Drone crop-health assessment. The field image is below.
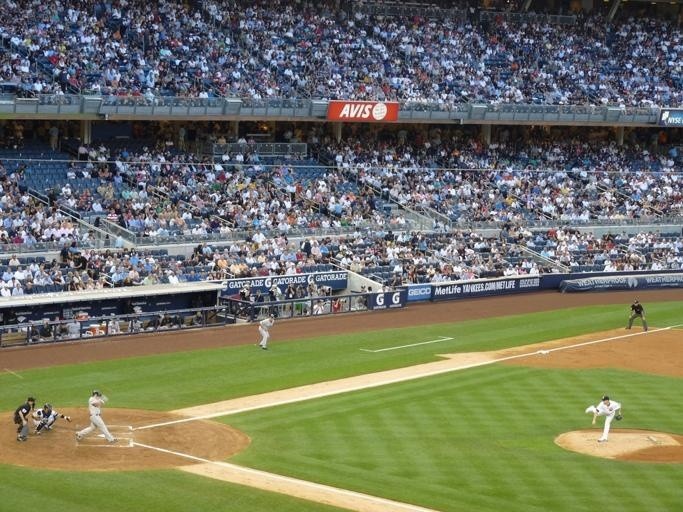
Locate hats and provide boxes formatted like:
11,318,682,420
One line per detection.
602,396,609,400
634,299,639,303
271,314,275,318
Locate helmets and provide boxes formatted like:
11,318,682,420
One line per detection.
93,390,101,395
28,398,35,401
44,403,52,415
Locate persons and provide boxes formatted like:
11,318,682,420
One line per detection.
14,397,35,442
585,395,621,441
259,313,274,349
32,403,71,435
75,391,117,442
1,1,683,338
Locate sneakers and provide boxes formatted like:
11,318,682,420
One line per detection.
44,425,52,431
598,439,608,441
262,348,267,350
643,329,647,332
76,432,82,441
259,345,263,347
586,405,594,413
106,439,118,444
625,327,631,329
17,436,27,441
33,430,41,436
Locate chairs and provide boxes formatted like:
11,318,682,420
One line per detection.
0,143,399,241
